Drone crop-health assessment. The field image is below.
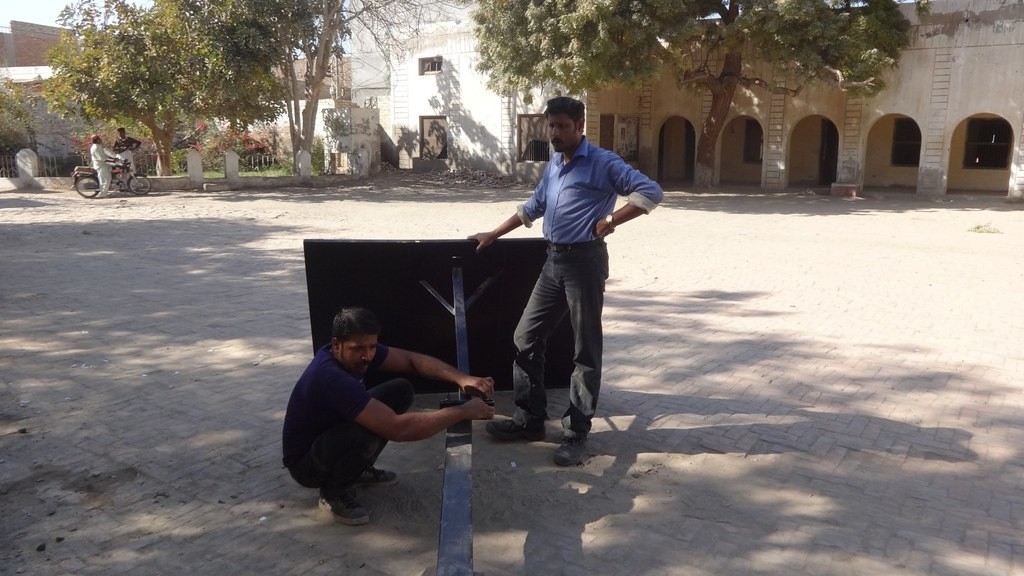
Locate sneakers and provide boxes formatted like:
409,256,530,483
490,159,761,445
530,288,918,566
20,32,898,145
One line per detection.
485,420,546,442
354,465,399,487
554,434,586,466
320,485,370,525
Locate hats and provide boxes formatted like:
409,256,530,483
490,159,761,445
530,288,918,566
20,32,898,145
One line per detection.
90,134,99,140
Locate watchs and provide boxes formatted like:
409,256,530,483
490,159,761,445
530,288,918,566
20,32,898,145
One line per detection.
606,213,616,228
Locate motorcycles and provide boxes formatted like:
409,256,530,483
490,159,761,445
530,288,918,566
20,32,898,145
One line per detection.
72,157,151,198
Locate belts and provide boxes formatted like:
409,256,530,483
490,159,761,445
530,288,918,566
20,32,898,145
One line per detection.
549,240,599,250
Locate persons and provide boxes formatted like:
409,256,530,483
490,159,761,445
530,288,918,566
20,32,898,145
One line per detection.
89,135,119,198
111,127,142,192
283,305,497,527
466,96,664,465
